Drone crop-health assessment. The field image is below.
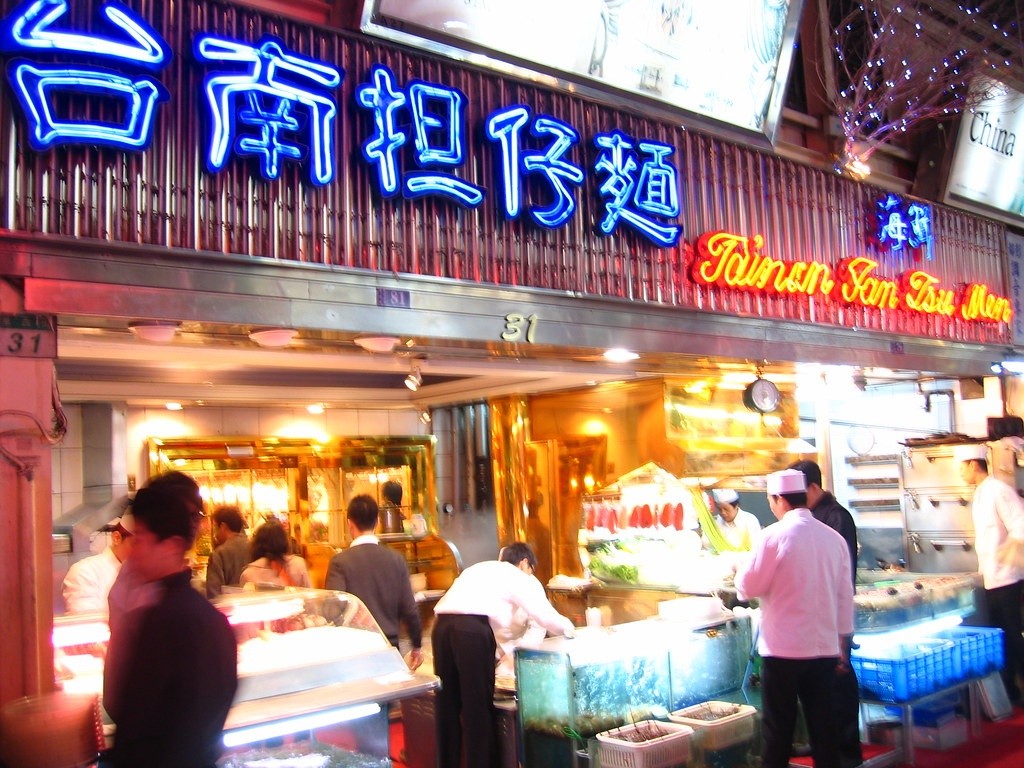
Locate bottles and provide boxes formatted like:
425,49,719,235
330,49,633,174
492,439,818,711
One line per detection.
411,514,427,538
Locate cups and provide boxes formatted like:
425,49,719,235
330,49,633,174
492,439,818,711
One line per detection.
402,520,411,534
586,608,602,632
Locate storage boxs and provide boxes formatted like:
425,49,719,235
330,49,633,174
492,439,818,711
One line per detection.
853,570,1007,701
515,614,760,768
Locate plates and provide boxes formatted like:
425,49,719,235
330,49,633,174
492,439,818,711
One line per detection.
374,533,413,542
421,590,445,600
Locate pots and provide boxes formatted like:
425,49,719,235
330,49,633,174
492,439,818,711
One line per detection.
0,692,98,768
378,509,404,533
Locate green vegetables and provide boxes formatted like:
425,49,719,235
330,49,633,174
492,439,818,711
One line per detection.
588,540,637,583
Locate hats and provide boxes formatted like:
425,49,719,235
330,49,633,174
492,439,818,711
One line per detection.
767,470,806,495
712,489,740,505
121,505,136,536
956,445,988,461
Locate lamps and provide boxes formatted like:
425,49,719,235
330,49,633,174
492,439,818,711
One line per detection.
405,355,426,392
990,361,1004,374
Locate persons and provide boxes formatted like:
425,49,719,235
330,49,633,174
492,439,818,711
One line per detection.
325,495,422,659
432,541,577,768
381,482,402,507
733,469,864,768
701,488,761,554
955,445,1024,709
239,520,310,590
785,460,858,662
62,505,133,616
206,508,253,599
99,470,238,768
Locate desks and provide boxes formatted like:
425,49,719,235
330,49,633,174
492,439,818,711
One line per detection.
859,672,995,768
